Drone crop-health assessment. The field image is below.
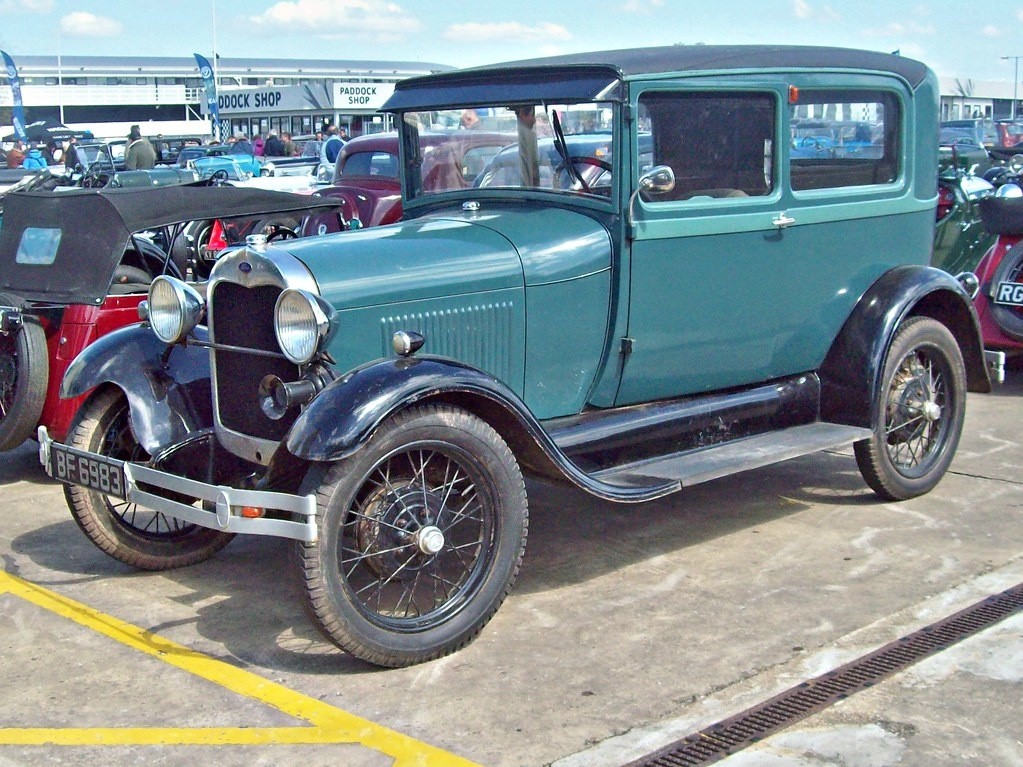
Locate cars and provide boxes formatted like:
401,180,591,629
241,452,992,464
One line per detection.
36,45,1007,670
0,115,1023,455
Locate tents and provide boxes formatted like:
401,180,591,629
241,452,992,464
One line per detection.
1,119,92,144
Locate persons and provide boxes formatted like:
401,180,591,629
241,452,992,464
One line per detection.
42,137,77,165
6,140,47,168
154,134,164,152
124,125,156,171
209,129,299,156
315,125,351,180
536,114,552,138
463,110,500,176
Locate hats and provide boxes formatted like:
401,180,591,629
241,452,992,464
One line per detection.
126,132,141,140
